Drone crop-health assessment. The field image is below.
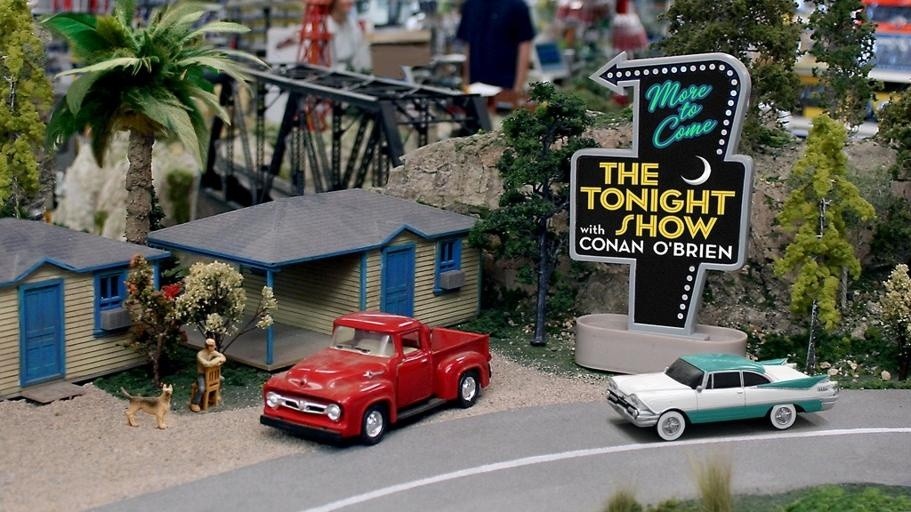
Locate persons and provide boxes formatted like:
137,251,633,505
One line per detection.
300,0,373,115
189,337,226,412
450,0,538,140
